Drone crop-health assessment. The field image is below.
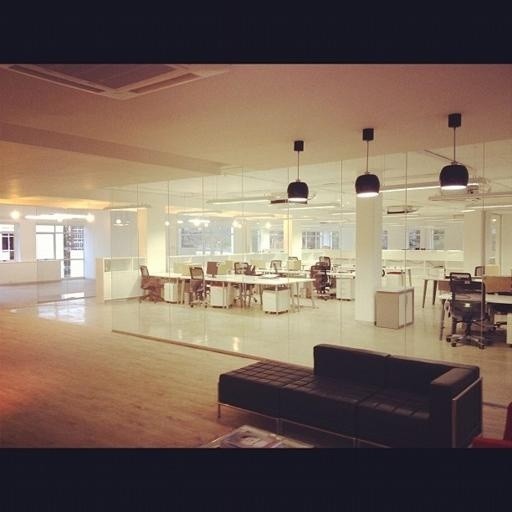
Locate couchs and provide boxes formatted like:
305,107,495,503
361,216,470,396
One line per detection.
208,339,486,452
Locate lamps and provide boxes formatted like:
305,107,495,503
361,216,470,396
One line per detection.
100,107,512,223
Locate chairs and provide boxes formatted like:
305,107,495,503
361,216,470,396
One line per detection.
133,249,511,350
